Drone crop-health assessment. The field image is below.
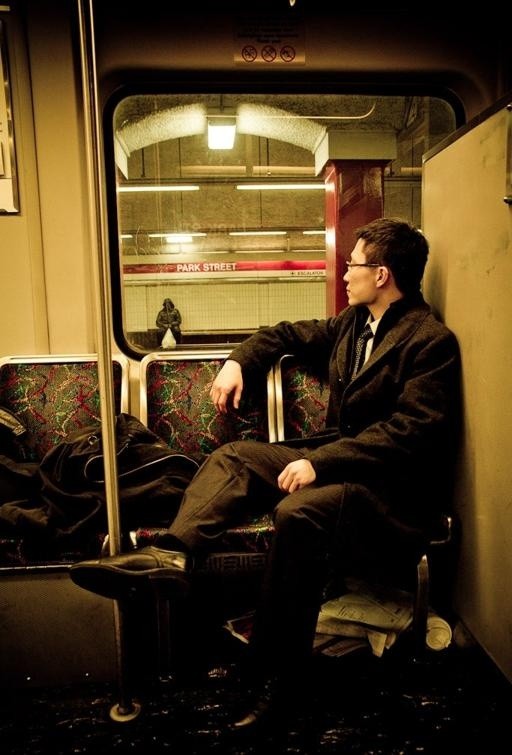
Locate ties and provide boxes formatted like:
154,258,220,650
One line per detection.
349,325,372,380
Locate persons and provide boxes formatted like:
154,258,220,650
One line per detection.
156,298,182,347
69,220,465,737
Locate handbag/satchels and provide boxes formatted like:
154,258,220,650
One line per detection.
27,413,201,535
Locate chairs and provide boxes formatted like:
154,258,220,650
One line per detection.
0,347,460,713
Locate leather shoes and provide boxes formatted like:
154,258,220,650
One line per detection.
68,546,195,602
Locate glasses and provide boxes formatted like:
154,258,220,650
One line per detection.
345,260,382,271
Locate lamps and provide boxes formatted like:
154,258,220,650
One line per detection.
205,115,236,151
